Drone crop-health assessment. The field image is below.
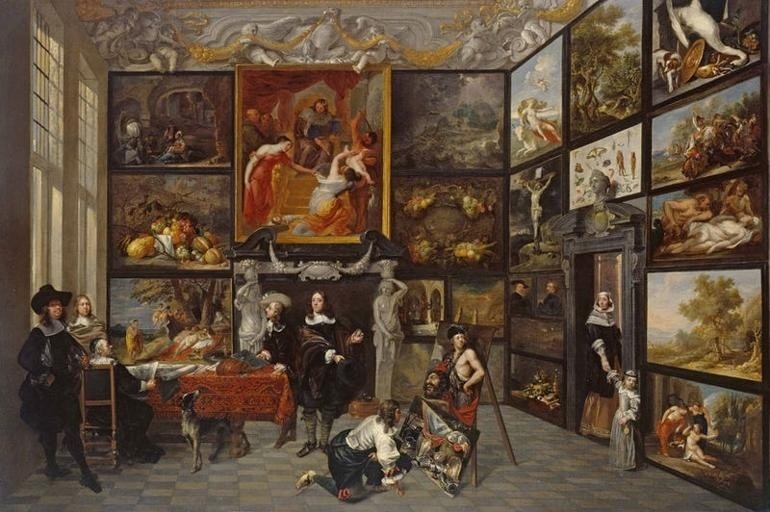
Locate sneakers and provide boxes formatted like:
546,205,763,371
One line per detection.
293,470,317,488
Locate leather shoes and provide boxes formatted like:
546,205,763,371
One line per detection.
295,441,331,459
80,473,102,492
44,461,59,478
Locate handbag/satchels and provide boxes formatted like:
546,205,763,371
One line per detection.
19,376,59,430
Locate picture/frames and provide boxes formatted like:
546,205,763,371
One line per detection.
102,60,505,407
508,0,770,511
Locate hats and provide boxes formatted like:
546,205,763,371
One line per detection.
445,324,470,338
31,285,72,313
260,291,293,310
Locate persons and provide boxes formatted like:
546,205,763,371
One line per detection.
0,0,767,506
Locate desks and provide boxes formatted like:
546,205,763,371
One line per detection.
114,356,296,460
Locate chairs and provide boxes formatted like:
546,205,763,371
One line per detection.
79,360,123,471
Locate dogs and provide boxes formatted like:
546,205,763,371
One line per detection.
175,389,237,475
